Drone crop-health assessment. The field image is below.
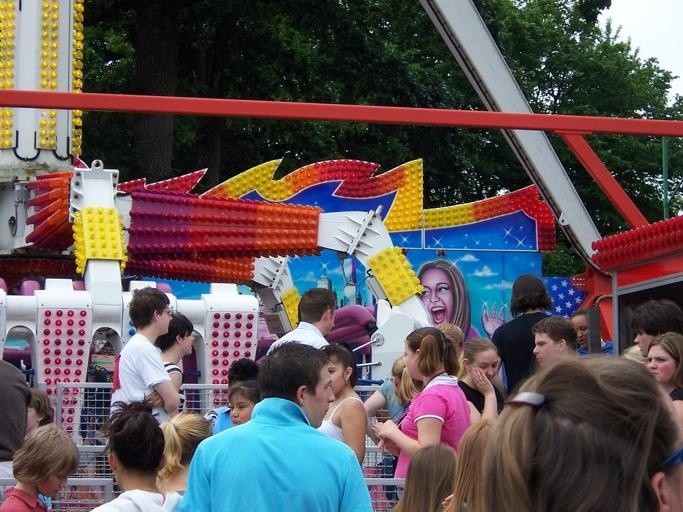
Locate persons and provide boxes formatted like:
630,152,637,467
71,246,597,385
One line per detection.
364,276,682,512
0,356,32,501
177,288,373,512
87,392,212,512
109,287,195,425
0,421,81,512
25,389,54,441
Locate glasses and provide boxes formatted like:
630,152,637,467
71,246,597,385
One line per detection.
163,310,171,316
